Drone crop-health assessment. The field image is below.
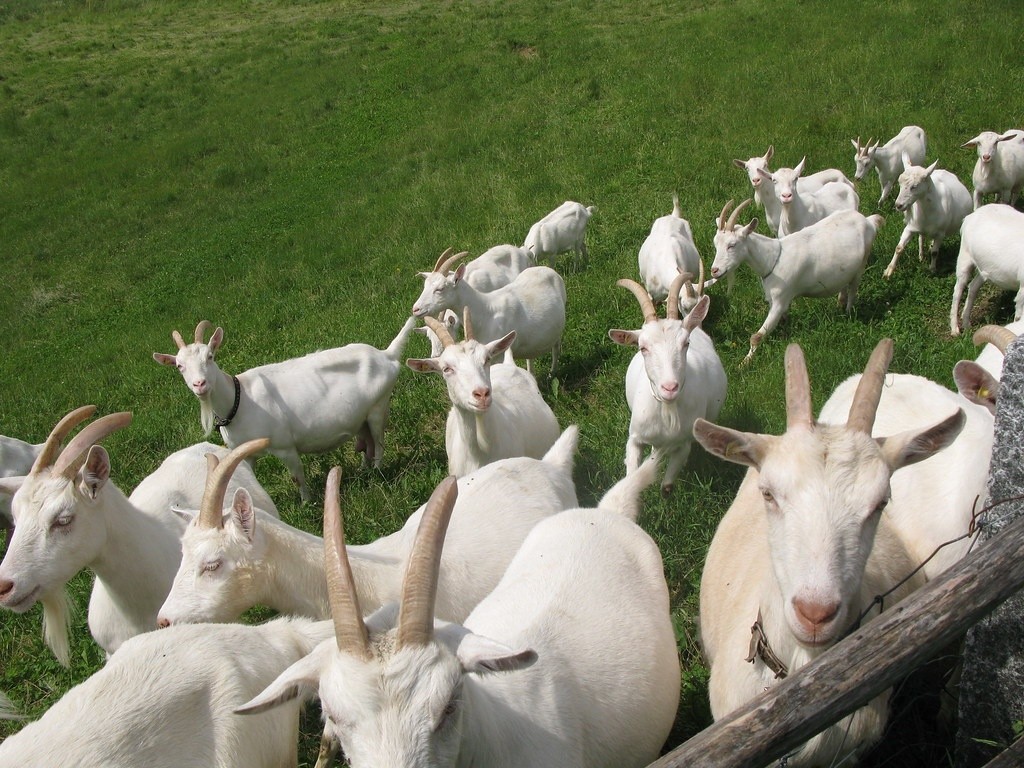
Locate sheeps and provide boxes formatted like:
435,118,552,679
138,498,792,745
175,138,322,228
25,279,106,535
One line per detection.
0,125,1024,768
